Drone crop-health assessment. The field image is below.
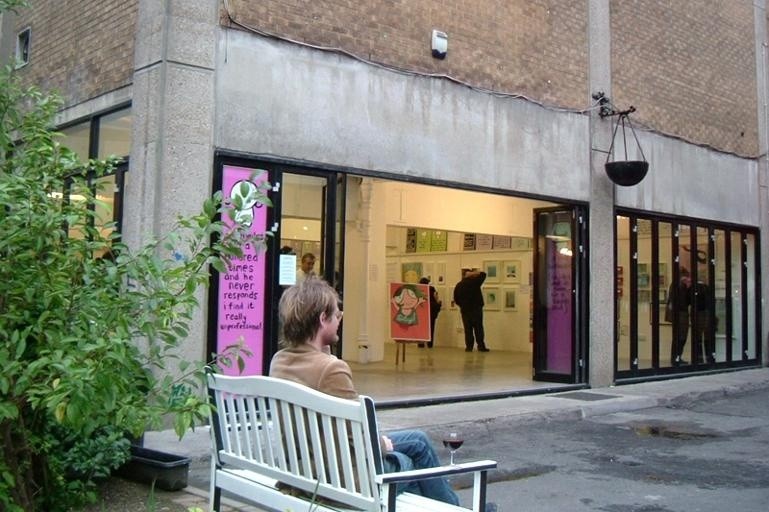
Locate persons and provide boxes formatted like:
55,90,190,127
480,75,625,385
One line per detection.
417,278,441,348
269,279,498,512
296,253,316,286
453,271,490,351
669,267,689,367
689,274,716,364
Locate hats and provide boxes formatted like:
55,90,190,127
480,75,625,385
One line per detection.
420,277,430,283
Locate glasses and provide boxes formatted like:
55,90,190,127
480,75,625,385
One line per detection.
326,310,344,320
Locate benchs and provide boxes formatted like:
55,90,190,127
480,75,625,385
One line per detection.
203,365,497,511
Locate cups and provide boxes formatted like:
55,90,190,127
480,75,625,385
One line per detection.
442,430,463,472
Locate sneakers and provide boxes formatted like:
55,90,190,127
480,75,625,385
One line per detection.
471,502,502,512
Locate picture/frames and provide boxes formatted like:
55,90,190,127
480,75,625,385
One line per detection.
501,259,522,284
649,303,673,324
659,261,668,303
425,261,435,285
416,228,433,254
476,233,492,251
482,286,502,311
450,286,457,310
463,232,476,251
388,281,431,342
493,235,510,249
637,274,650,289
637,290,651,303
402,262,422,283
504,288,519,312
431,231,447,252
678,242,711,288
637,262,649,274
511,237,529,250
280,238,320,260
714,296,736,339
405,228,417,253
437,261,448,287
482,260,500,283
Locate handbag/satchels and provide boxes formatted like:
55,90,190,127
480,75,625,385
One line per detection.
665,286,672,322
